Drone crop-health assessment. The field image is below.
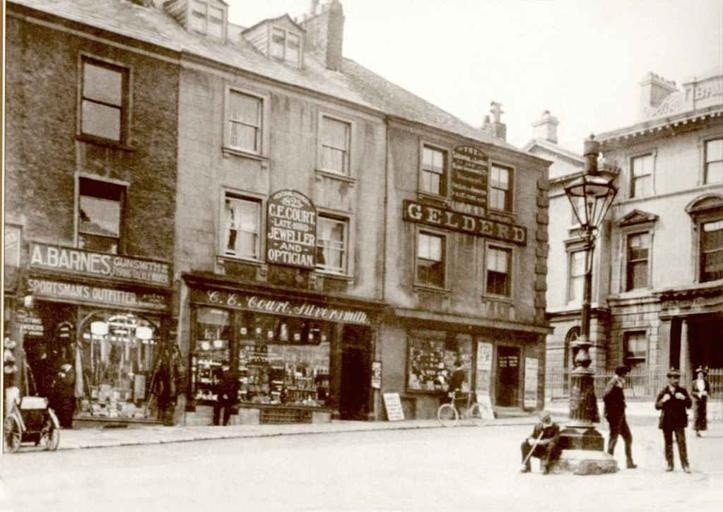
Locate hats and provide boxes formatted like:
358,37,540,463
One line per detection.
694,364,708,375
667,367,681,377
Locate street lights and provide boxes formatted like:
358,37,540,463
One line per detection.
559,139,618,450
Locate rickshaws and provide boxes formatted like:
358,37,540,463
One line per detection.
4,395,62,453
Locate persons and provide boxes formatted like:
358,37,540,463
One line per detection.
656,372,692,473
208,360,237,425
55,359,76,428
691,369,711,437
521,410,561,474
602,366,637,469
440,361,465,406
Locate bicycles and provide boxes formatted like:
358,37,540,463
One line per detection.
437,388,489,427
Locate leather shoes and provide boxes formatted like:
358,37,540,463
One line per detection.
683,466,690,473
627,462,637,467
665,465,673,471
